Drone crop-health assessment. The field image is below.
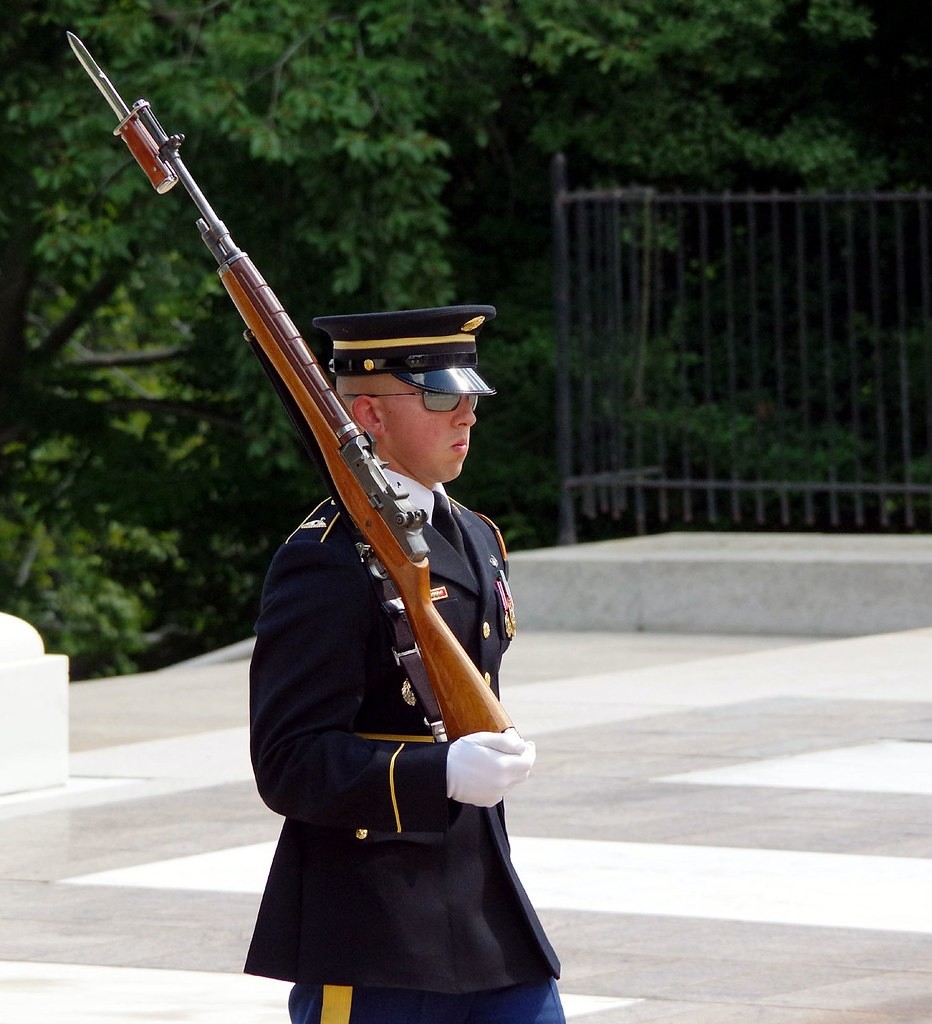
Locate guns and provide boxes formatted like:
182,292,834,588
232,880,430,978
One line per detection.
66,27,516,748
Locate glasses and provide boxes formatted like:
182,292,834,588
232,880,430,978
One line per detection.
341,391,478,412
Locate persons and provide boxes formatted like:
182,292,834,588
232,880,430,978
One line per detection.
243,307,565,1024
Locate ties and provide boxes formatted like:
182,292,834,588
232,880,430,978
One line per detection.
431,490,467,564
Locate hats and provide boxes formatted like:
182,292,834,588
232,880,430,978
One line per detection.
312,305,497,396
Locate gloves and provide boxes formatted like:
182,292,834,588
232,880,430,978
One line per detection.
445,730,536,806
